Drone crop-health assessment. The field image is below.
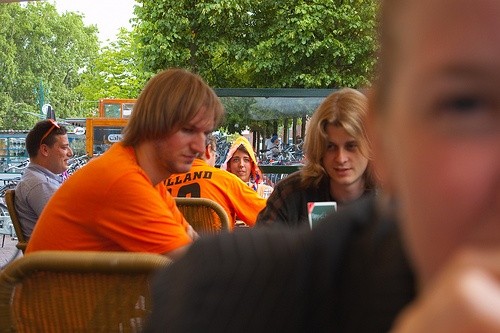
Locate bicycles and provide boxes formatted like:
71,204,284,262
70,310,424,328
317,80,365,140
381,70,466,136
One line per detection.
0,135,304,268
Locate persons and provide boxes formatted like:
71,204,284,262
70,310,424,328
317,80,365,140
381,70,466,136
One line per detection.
23,68,224,263
261,133,304,160
144,0,500,333
220,136,275,201
161,135,267,229
255,88,379,227
15,119,74,243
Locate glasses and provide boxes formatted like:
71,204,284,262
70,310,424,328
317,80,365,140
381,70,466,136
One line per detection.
39,117,60,144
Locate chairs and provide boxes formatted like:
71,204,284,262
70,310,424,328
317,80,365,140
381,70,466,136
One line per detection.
174,199,230,231
0,252,171,333
6,189,27,255
